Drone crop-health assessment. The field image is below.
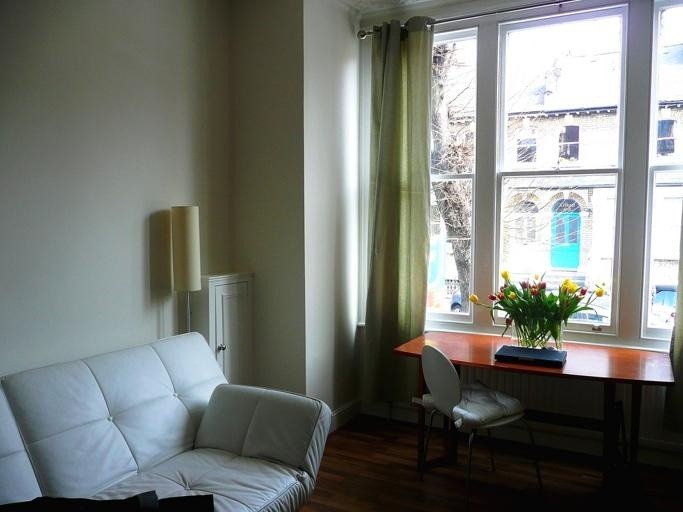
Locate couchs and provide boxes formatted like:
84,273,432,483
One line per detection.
0,330,333,512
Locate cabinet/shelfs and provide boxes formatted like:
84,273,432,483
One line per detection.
191,272,253,386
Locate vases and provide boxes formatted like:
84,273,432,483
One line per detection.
515,316,564,352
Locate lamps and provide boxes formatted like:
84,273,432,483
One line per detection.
171,205,202,332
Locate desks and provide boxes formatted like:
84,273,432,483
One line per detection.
394,330,675,491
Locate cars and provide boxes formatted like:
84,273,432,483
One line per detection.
450,281,677,330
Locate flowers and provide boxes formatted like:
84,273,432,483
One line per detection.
469,272,604,352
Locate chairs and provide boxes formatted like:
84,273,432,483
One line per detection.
421,345,544,501
